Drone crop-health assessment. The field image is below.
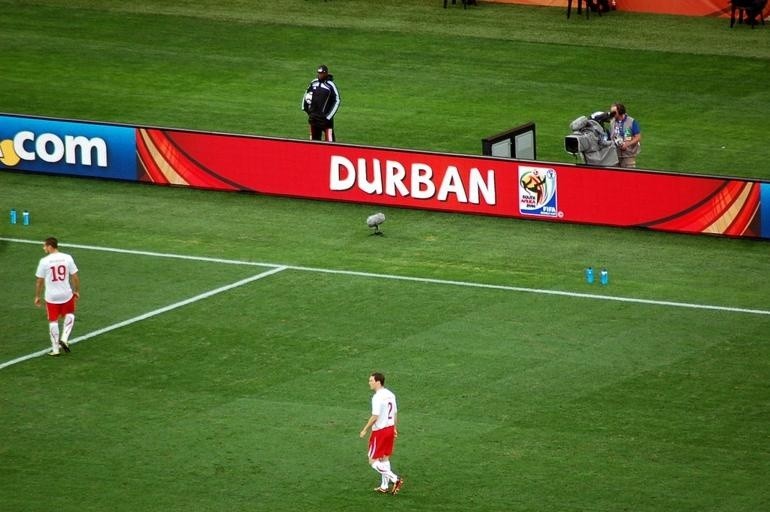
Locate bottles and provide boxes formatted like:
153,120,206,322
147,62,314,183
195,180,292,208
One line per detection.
10,208,17,224
600,268,608,286
23,210,30,225
586,267,594,283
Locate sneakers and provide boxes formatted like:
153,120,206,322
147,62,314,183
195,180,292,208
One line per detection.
59,339,71,353
391,476,404,496
374,487,390,494
47,351,60,356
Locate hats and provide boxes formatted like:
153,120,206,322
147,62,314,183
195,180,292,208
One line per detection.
317,65,328,73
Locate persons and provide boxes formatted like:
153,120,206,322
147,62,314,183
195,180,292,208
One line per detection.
359,374,404,493
35,237,80,356
610,101,642,169
301,64,340,143
